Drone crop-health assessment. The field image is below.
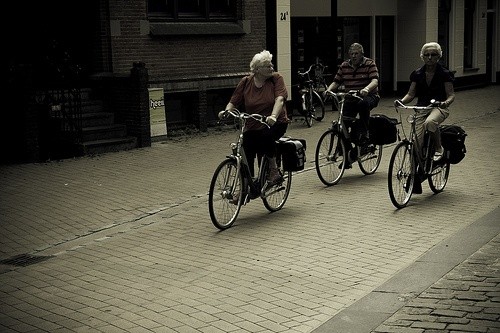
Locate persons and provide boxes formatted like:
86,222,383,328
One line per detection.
322,43,380,169
394,42,454,194
217,49,288,204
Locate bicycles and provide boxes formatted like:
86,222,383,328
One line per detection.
315,90,401,186
387,97,468,209
209,108,306,230
295,63,325,128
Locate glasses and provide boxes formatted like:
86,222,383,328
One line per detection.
423,52,439,58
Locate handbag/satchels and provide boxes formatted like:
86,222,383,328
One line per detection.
440,125,467,165
338,160,356,170
369,112,398,145
282,138,306,172
406,184,422,194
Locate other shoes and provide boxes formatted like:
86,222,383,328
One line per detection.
271,169,284,182
229,194,250,206
